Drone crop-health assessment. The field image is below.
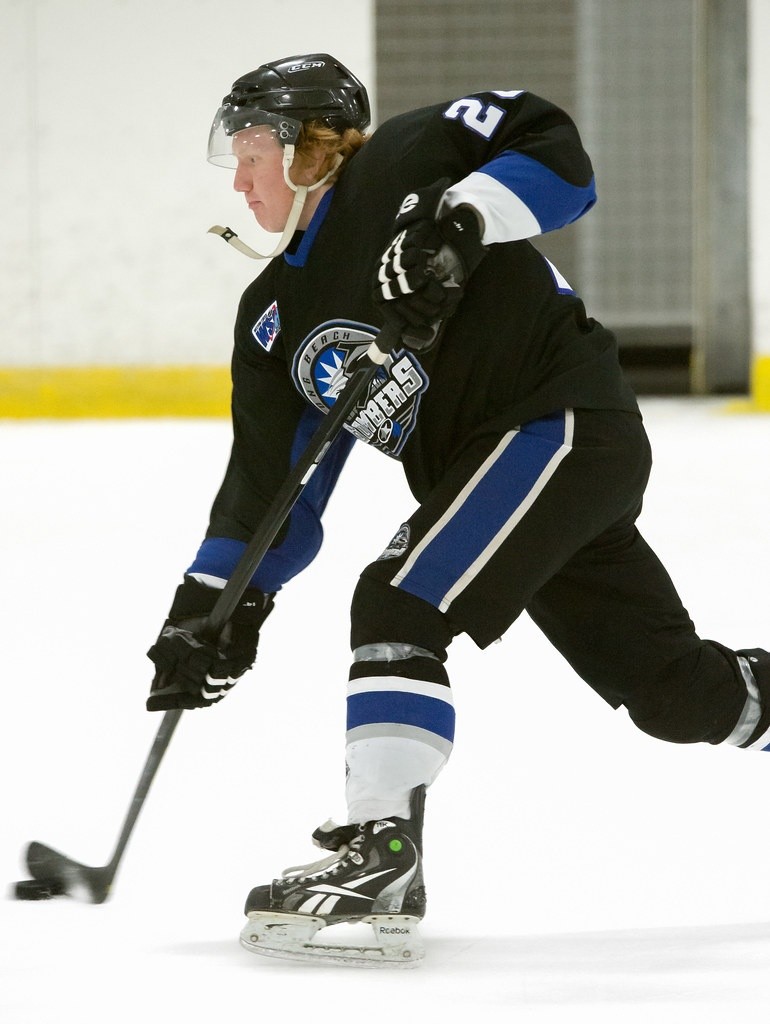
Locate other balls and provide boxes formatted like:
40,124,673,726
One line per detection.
15,880,51,900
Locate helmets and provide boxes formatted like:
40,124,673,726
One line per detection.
221,53,371,149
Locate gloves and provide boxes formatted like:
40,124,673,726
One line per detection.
144,574,282,711
372,208,490,355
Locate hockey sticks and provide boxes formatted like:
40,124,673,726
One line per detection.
27,260,455,913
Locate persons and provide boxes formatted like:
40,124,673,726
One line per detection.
149,55,769,971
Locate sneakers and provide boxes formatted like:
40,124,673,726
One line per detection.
239,784,426,970
737,647,770,753
